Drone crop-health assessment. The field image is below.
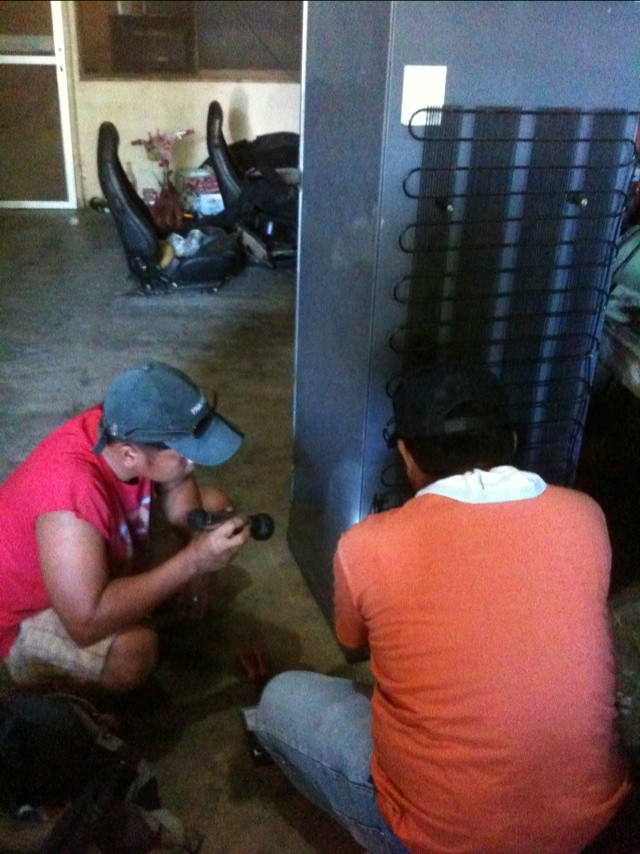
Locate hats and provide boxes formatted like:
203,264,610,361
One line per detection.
93,361,245,466
387,348,509,448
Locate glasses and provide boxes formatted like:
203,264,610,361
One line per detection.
124,385,218,439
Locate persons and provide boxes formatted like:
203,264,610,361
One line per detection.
0,362,252,735
253,355,632,853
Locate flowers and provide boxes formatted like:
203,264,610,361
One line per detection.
133,129,196,187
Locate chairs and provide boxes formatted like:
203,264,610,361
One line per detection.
206,101,299,268
95,120,245,295
